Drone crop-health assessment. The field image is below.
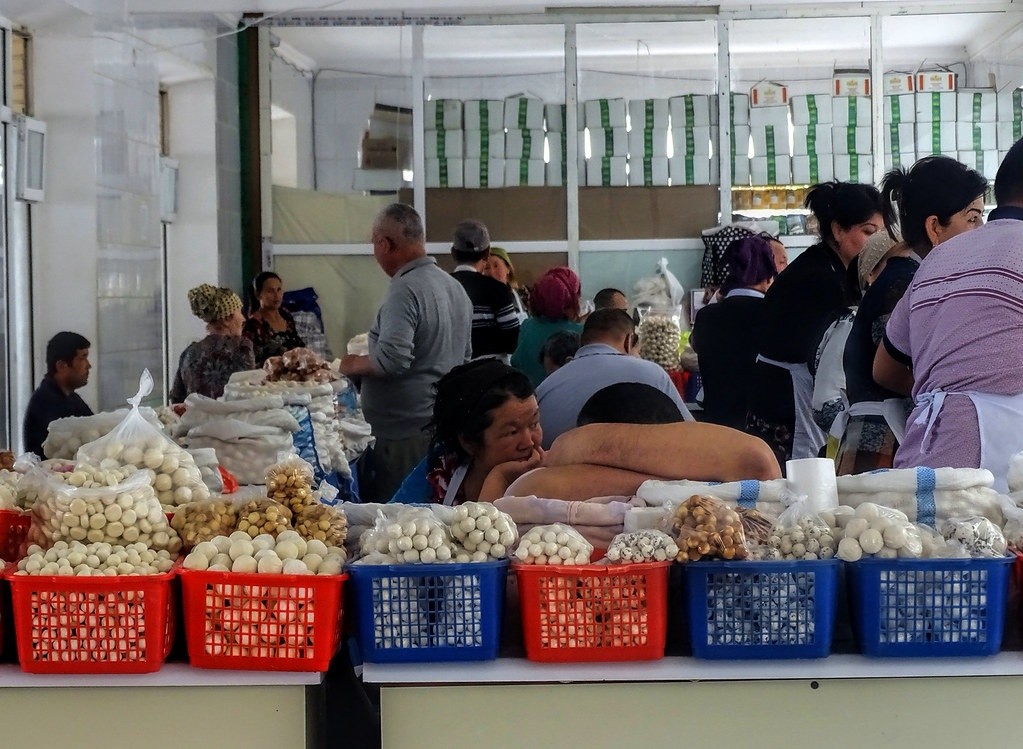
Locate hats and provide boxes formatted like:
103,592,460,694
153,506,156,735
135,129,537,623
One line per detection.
530,266,581,317
491,247,516,281
451,221,490,261
189,284,244,322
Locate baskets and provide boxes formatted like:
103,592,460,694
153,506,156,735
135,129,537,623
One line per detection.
179,549,348,673
344,545,511,664
0,511,31,562
681,556,843,660
850,548,1017,658
512,552,674,665
3,554,186,675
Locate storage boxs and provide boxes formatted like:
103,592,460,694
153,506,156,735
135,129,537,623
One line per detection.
0,57,1023,673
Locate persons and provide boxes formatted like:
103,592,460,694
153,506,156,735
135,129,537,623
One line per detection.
745,182,896,479
835,156,991,475
339,203,474,504
506,382,782,503
388,357,543,506
170,285,256,403
446,220,528,366
873,137,1023,495
512,267,628,388
534,308,695,452
689,236,787,431
23,332,94,461
241,271,305,369
